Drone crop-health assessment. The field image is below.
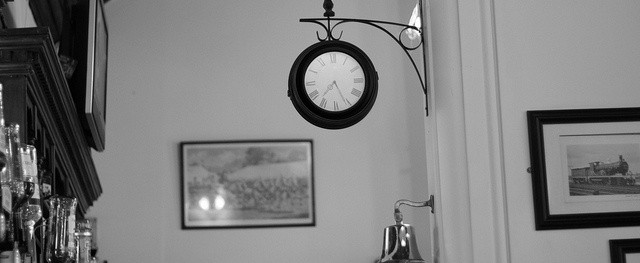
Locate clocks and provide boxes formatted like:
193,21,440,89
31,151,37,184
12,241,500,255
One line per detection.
288,39,379,129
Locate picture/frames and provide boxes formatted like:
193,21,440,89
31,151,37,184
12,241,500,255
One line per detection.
180,139,315,228
526,106,640,230
608,238,640,263
